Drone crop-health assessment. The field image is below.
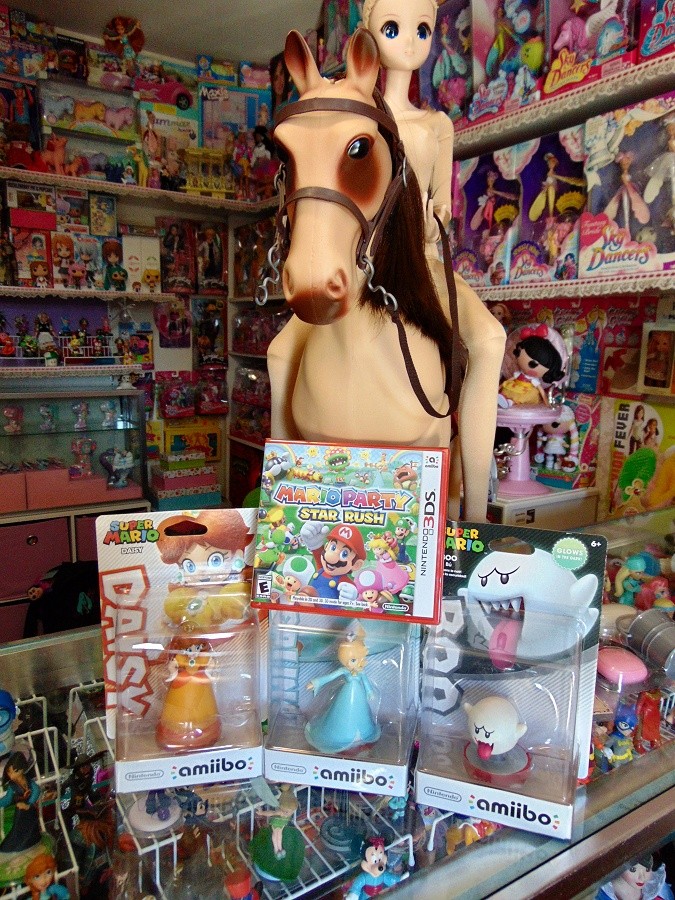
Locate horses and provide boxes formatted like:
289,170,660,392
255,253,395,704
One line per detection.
262,26,466,524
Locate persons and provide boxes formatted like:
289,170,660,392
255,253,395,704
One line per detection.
0,0,675,899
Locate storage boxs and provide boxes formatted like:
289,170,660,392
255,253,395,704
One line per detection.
404,0,675,521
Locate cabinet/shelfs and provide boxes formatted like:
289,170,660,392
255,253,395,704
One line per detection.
451,35,675,529
1,162,302,517
1,504,157,644
